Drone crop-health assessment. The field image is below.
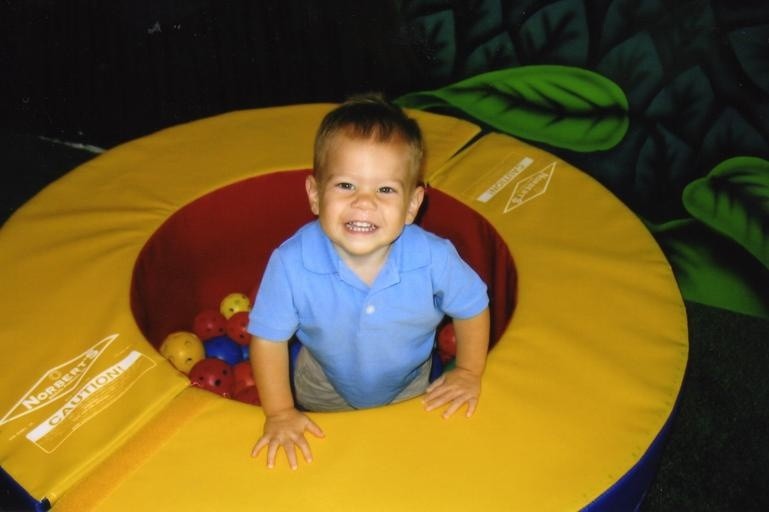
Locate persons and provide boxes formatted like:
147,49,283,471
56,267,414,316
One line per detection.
248,90,494,474
